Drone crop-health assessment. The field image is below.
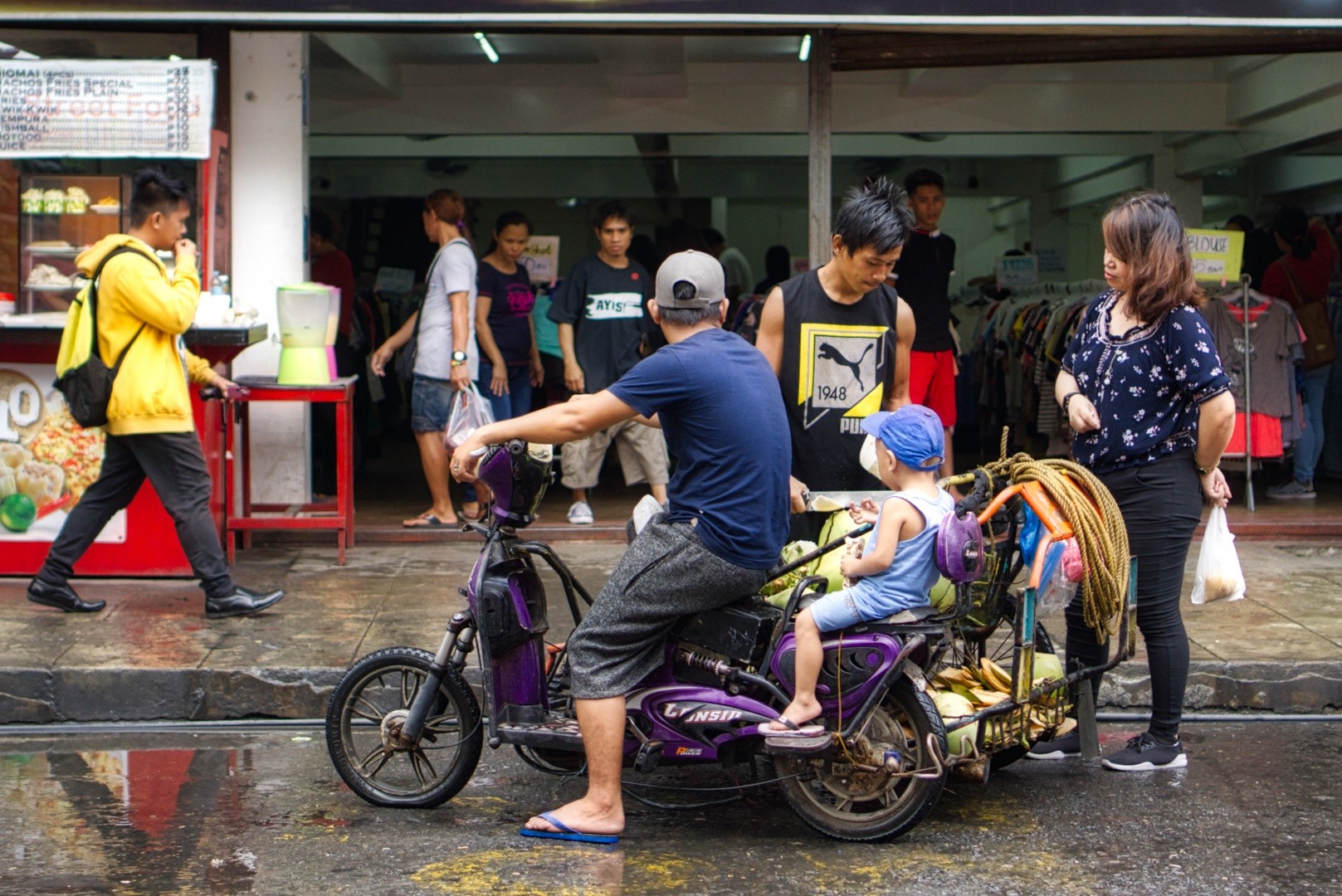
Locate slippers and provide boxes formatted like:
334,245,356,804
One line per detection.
459,499,499,523
518,809,621,845
756,715,826,739
403,512,460,529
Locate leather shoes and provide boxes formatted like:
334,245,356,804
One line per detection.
26,576,107,613
204,585,286,620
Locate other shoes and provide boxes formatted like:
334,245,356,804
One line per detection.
567,501,594,524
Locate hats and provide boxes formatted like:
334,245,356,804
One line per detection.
859,405,946,471
654,249,727,310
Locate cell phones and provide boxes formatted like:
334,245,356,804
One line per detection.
218,385,250,400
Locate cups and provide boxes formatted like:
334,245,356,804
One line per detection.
20,195,88,215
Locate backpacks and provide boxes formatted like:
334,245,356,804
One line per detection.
52,249,163,432
1276,259,1336,370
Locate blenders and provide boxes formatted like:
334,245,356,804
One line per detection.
315,282,341,383
277,282,330,385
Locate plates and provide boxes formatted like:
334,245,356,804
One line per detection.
26,246,92,253
90,203,121,214
24,282,87,290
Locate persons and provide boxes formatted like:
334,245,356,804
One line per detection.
449,249,792,844
1223,213,1342,499
25,168,285,619
46,747,227,893
757,404,955,738
755,176,915,514
1025,191,1237,772
311,168,965,529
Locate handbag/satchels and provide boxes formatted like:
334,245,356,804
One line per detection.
1191,505,1247,606
393,241,475,380
440,380,496,479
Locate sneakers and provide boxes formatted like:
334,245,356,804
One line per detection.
1025,729,1102,760
1265,478,1317,500
1100,731,1188,772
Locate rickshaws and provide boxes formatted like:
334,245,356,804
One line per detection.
321,438,1137,848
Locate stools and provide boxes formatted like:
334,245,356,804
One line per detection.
226,375,359,566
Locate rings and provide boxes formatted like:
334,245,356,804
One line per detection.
454,465,458,470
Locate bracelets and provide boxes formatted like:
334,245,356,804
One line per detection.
1063,392,1084,414
1195,460,1220,475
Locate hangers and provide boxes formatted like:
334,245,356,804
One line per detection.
996,274,1293,317
